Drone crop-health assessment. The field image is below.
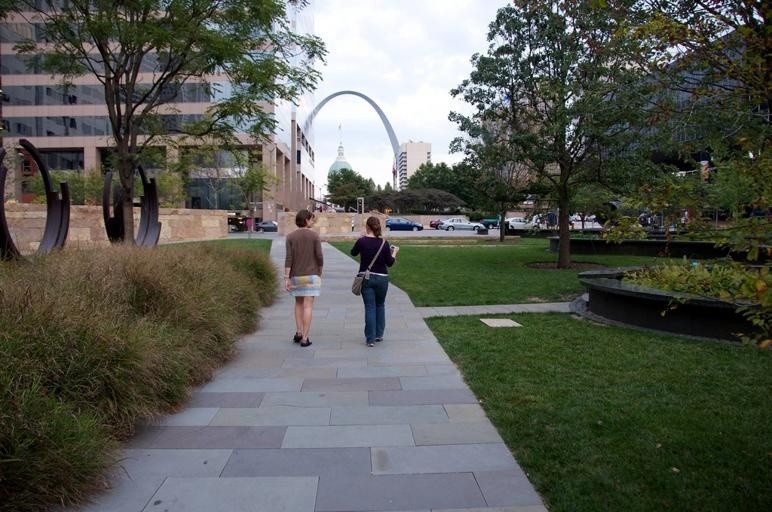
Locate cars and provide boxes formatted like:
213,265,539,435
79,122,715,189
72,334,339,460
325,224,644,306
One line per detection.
437,218,486,231
430,217,449,229
256,220,277,232
569,213,597,223
505,217,542,235
385,217,423,231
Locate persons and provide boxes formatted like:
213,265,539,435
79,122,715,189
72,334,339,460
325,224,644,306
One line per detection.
246,216,253,233
282,210,323,346
350,215,400,347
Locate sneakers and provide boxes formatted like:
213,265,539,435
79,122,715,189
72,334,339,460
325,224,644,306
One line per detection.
367,337,383,347
294,335,303,343
300,337,312,347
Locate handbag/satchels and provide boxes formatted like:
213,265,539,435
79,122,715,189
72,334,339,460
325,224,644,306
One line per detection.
351,277,364,296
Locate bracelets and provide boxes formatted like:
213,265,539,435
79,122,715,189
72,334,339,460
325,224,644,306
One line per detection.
284,274,289,278
